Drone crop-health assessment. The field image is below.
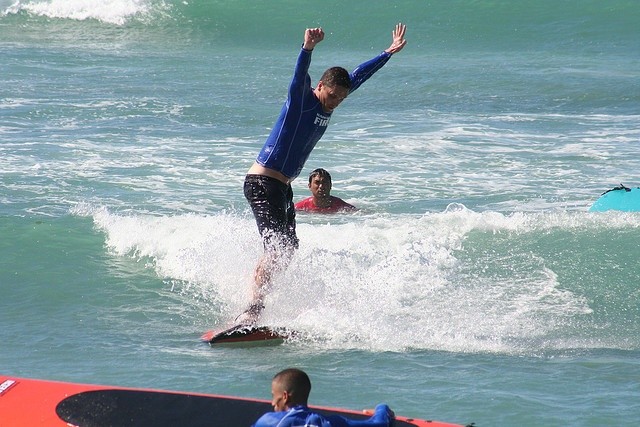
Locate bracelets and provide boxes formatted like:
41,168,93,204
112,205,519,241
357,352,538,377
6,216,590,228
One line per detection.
301,43,314,52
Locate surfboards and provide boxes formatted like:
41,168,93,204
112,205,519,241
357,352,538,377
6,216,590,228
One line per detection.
0,377,465,427
589,183,640,213
202,324,284,349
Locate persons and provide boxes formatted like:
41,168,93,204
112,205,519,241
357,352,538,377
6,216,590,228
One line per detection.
243,23,407,321
251,368,392,426
294,168,359,214
